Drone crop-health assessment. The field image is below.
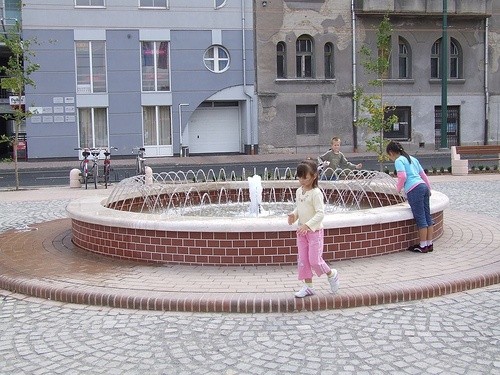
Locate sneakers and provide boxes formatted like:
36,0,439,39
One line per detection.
428,244,433,252
294,285,315,298
329,269,339,292
408,245,428,253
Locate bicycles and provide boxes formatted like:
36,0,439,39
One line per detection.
101,147,118,189
74,148,90,190
90,148,101,189
132,148,147,183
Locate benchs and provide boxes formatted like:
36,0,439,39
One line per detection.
451,145,500,176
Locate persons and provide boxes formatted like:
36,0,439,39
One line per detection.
386,141,434,254
287,157,339,298
318,137,362,181
